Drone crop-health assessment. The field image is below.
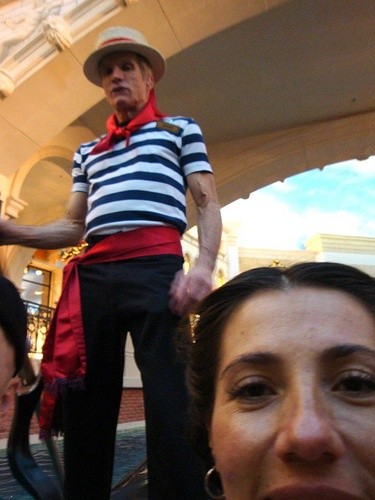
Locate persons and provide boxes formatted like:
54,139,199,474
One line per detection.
0,273,28,420
183,260,375,500
0,25,221,500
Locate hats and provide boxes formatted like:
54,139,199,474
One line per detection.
83,26,165,88
